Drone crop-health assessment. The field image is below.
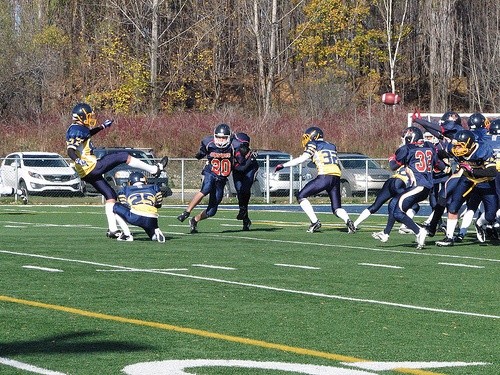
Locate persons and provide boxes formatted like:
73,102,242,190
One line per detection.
274,127,356,234
345,112,500,249
112,171,166,244
176,124,260,234
66,104,168,238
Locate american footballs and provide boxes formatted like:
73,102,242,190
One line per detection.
379,92,402,105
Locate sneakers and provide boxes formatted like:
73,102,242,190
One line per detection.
189,216,198,233
242,219,251,230
177,211,190,222
155,227,165,243
105,229,122,239
345,219,500,250
236,209,244,221
117,232,133,241
154,156,169,179
307,220,322,234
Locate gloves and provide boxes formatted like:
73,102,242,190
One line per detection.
460,162,473,174
388,157,395,162
75,159,86,166
195,153,204,159
103,120,112,127
274,164,284,172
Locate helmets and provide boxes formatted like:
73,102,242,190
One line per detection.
401,112,475,156
128,172,146,184
467,112,486,129
490,120,500,134
302,127,323,146
73,103,92,123
213,124,230,148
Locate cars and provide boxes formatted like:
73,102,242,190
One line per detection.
81,146,172,198
299,151,392,198
223,149,313,199
0,151,84,197
